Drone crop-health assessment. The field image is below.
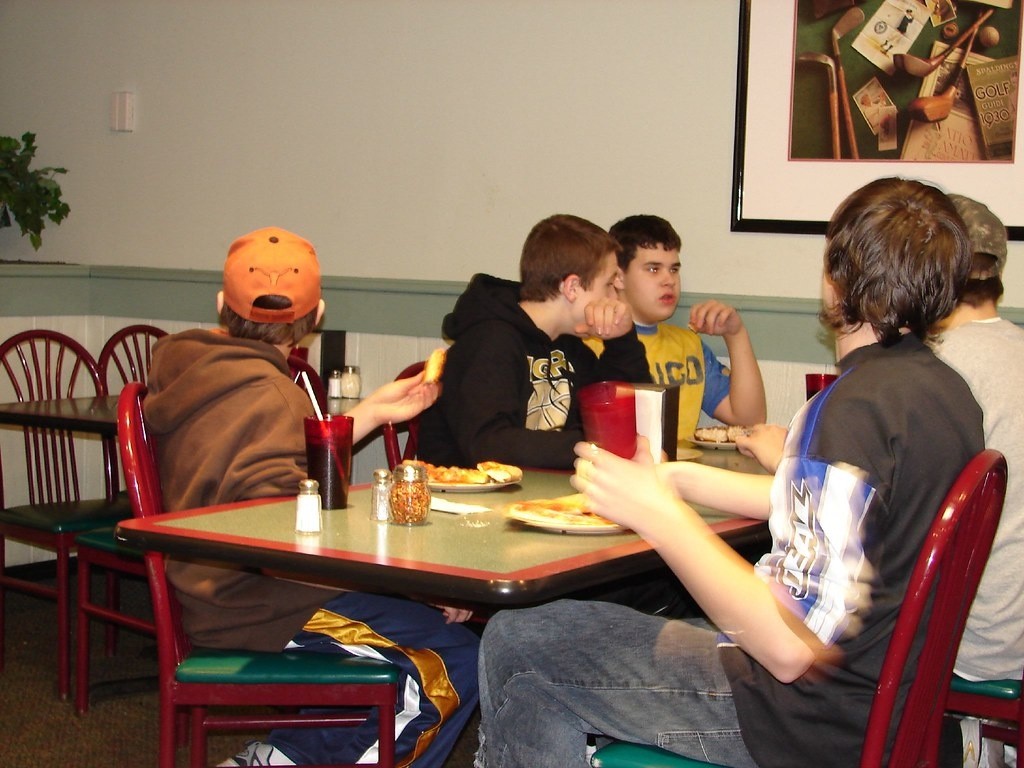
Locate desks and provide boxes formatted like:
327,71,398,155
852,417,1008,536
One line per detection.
3,394,363,436
114,445,774,609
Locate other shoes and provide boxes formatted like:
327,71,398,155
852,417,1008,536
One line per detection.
216,740,293,768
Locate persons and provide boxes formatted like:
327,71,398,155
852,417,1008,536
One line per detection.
141,226,481,768
415,215,687,616
578,214,767,448
471,177,986,768
880,9,915,54
735,193,1024,768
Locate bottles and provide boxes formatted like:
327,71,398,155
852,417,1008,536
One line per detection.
390,466,431,527
342,365,361,399
328,370,342,398
294,479,323,536
370,469,392,523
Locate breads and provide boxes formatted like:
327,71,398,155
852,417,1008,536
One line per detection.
694,425,752,443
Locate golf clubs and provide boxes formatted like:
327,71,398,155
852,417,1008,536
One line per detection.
906,8,984,122
893,8,994,78
830,6,866,159
798,50,842,159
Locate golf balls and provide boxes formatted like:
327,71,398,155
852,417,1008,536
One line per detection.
979,25,1001,48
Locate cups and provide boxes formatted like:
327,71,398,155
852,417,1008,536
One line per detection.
578,381,637,459
806,374,839,402
304,415,354,510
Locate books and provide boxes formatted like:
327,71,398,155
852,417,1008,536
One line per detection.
966,55,1018,159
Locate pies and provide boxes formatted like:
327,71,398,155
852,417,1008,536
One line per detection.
511,492,618,525
422,349,446,383
402,461,523,484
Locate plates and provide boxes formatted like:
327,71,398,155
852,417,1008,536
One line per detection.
684,436,739,450
428,482,519,493
677,447,703,461
509,517,630,533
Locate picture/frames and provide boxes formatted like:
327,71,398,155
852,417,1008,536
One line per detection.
730,1,1024,241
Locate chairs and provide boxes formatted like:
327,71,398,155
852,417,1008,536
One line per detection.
944,663,1024,768
1,317,436,768
590,448,1008,768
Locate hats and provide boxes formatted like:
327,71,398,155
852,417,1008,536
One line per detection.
948,194,1007,281
223,228,322,325
